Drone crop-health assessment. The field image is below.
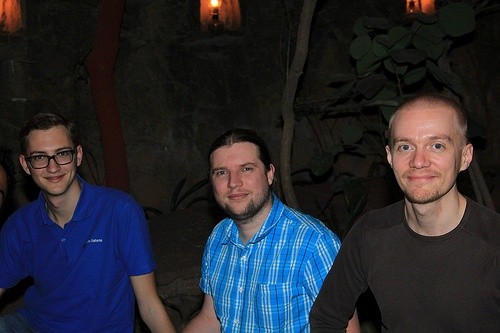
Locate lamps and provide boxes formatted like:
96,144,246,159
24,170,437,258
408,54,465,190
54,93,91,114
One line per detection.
207,0,225,32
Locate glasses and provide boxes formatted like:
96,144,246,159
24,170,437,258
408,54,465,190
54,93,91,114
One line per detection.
24,148,76,169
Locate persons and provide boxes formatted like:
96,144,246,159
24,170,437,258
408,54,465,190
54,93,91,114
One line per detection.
178,127,360,333
1,113,180,332
307,92,500,333
